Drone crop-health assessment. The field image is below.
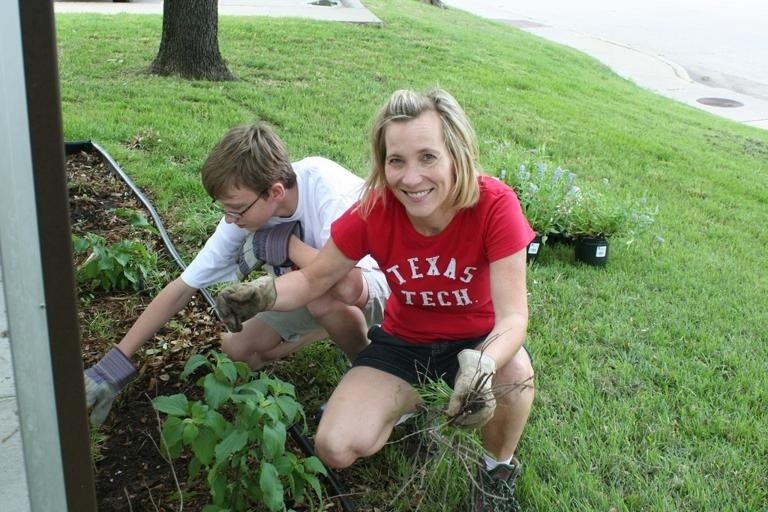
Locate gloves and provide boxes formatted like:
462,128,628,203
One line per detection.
236,220,303,282
218,274,279,332
444,347,497,429
82,348,139,428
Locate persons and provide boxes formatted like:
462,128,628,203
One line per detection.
216,85,537,509
79,122,416,436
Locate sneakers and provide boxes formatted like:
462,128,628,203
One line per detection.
400,403,439,467
473,454,521,511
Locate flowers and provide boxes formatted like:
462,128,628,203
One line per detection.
499,150,665,247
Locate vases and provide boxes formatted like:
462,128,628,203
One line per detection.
527,233,609,267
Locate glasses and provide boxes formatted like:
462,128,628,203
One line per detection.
210,192,261,219
